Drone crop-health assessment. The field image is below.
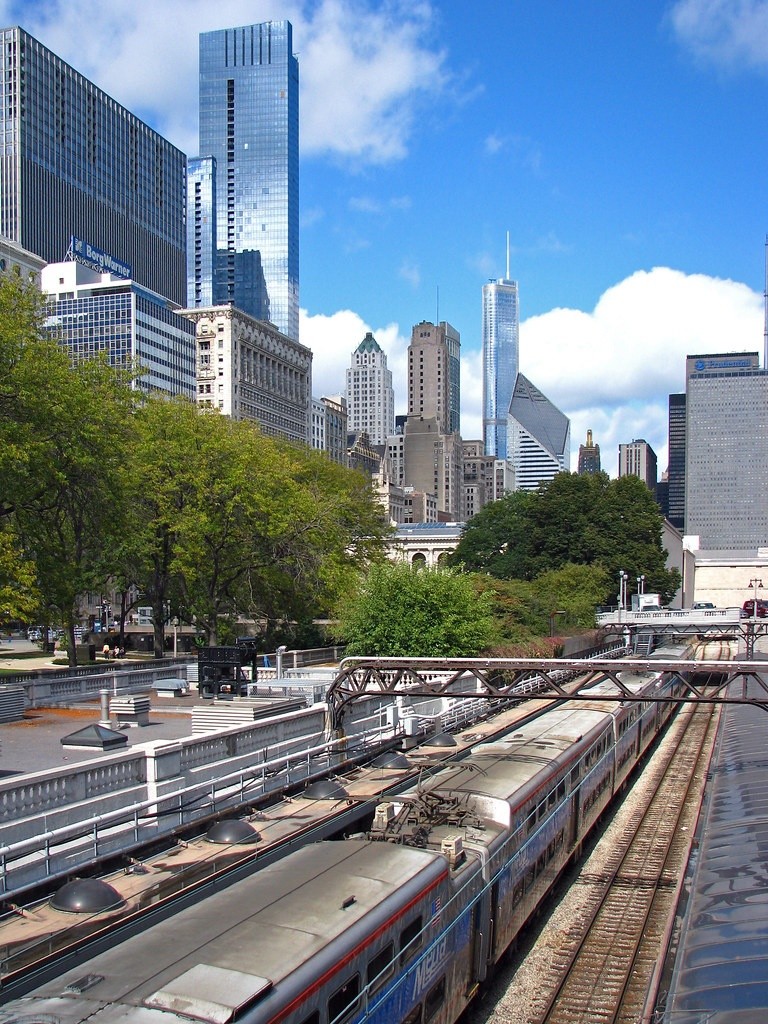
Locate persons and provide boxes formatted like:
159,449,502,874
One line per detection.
25,630,42,645
0,631,13,644
103,645,127,659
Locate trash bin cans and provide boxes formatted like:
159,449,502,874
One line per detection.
48,643,55,652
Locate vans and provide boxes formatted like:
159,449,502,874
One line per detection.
27,625,53,643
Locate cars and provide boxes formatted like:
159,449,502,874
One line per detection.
693,602,716,609
722,607,750,619
667,607,682,611
742,599,768,618
642,605,659,611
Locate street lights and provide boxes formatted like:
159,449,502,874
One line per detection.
641,575,645,595
623,574,628,610
619,570,624,610
637,577,641,594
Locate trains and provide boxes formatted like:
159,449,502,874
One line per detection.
649,651,767,1024
0,640,695,1024
